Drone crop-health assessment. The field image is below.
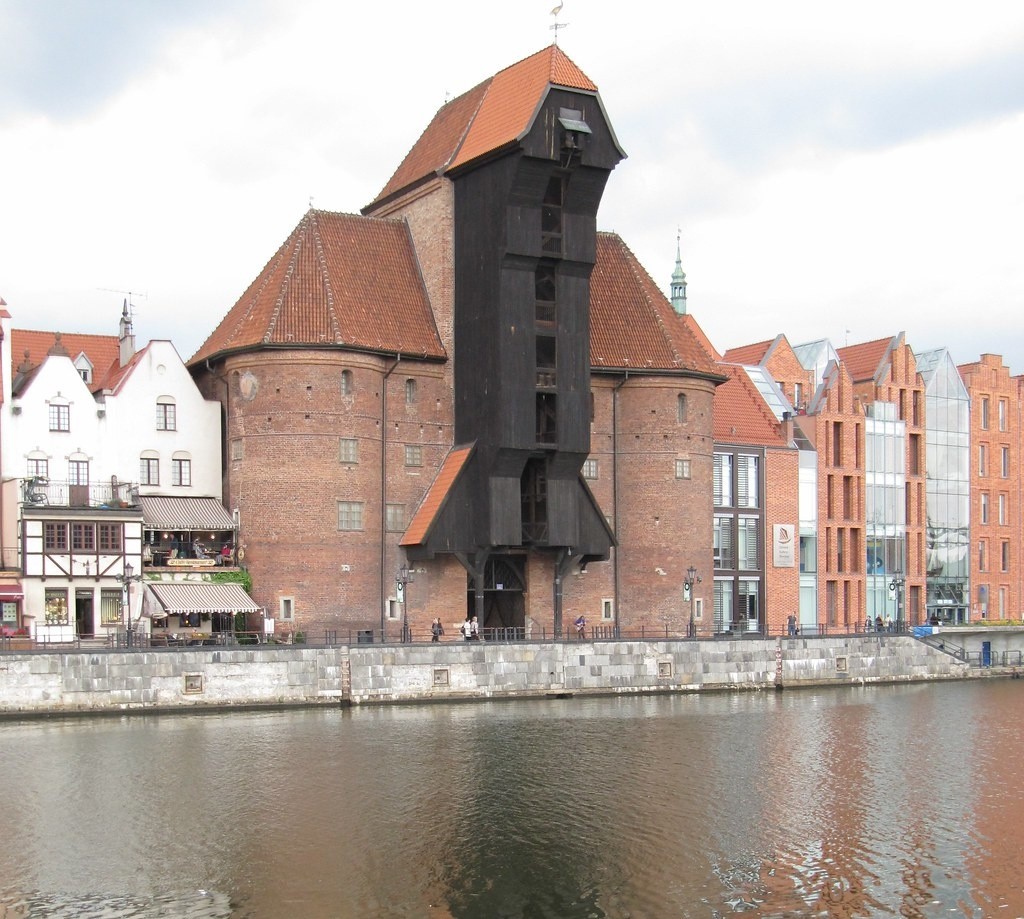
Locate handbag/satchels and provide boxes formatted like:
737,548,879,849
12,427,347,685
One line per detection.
889,620,894,626
470,631,476,636
574,623,583,632
430,629,436,634
461,628,465,633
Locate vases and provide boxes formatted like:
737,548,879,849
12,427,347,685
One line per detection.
120,502,128,509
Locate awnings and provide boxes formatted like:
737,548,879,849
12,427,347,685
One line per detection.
132,494,236,529
0,584,24,601
141,583,262,619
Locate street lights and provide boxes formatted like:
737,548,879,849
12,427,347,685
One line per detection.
683,562,702,637
396,563,416,642
890,568,906,633
115,561,143,648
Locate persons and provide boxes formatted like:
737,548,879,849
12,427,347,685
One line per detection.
169,533,231,566
191,626,199,646
431,617,443,643
786,611,799,636
143,544,155,567
930,612,950,626
462,617,479,640
574,614,586,638
865,614,902,632
